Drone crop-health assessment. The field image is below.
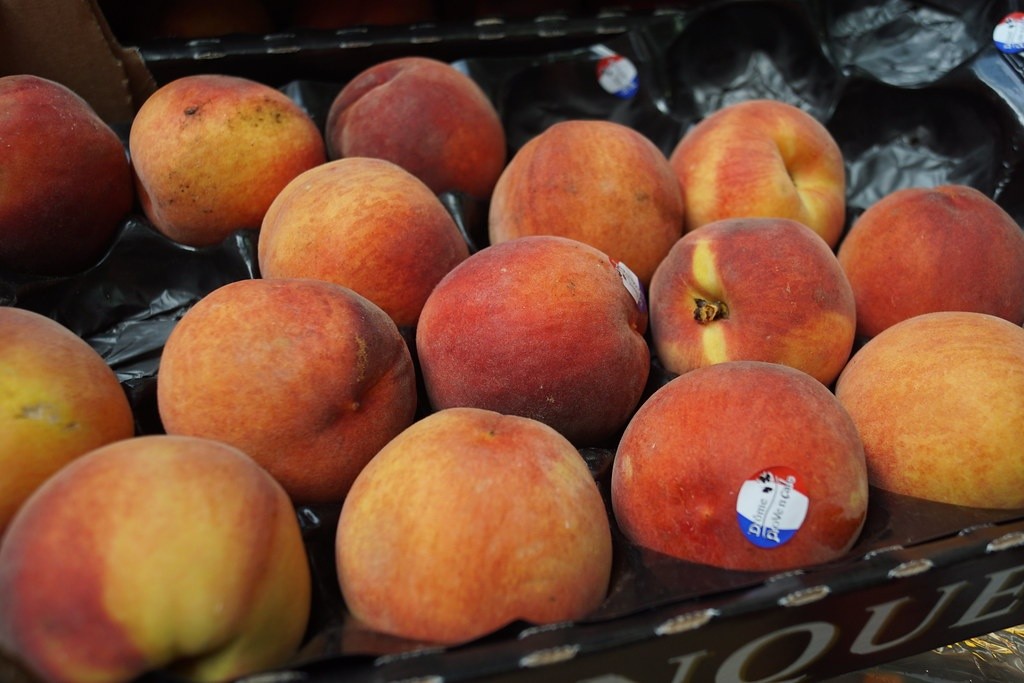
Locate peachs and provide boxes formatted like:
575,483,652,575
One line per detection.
0,57,1024,683
